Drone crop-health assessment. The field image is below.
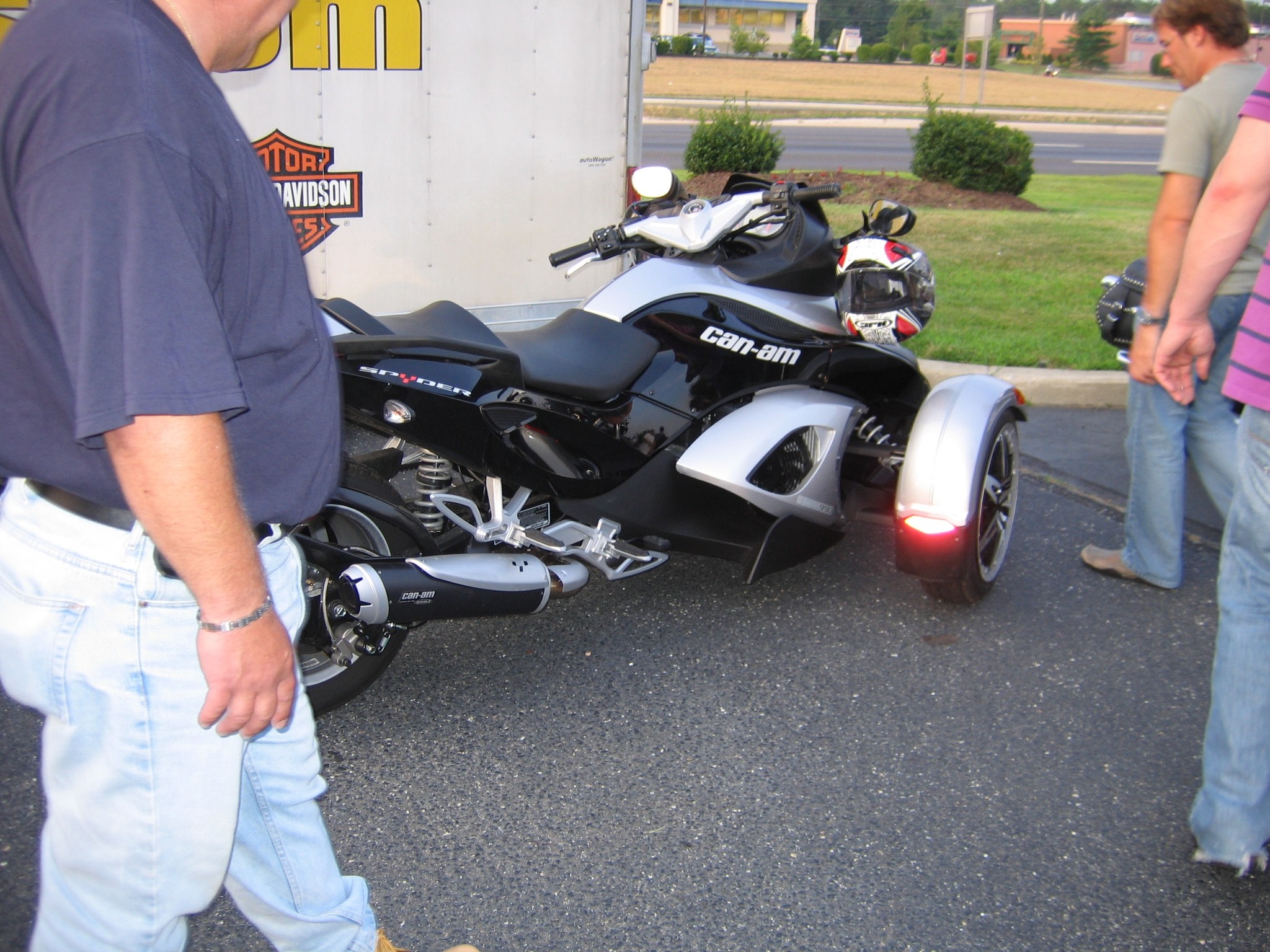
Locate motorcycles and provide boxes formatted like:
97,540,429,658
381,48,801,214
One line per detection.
277,168,1033,718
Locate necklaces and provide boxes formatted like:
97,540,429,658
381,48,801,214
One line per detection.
166,0,194,48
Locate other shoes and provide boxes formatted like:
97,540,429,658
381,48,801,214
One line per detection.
1082,544,1152,584
377,928,481,952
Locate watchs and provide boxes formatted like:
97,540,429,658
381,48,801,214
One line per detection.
1135,308,1166,327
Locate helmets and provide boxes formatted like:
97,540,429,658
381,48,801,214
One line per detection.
833,234,935,344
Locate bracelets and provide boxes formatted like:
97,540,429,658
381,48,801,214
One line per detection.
197,591,273,632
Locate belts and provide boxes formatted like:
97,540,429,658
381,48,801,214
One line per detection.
26,476,306,581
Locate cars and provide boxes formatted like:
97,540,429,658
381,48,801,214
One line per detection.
681,32,713,46
688,36,720,57
652,35,678,51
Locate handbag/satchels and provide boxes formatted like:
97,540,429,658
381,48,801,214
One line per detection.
1094,256,1152,350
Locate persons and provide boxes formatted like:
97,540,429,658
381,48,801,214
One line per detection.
0,0,478,952
1151,70,1270,874
1080,0,1270,588
